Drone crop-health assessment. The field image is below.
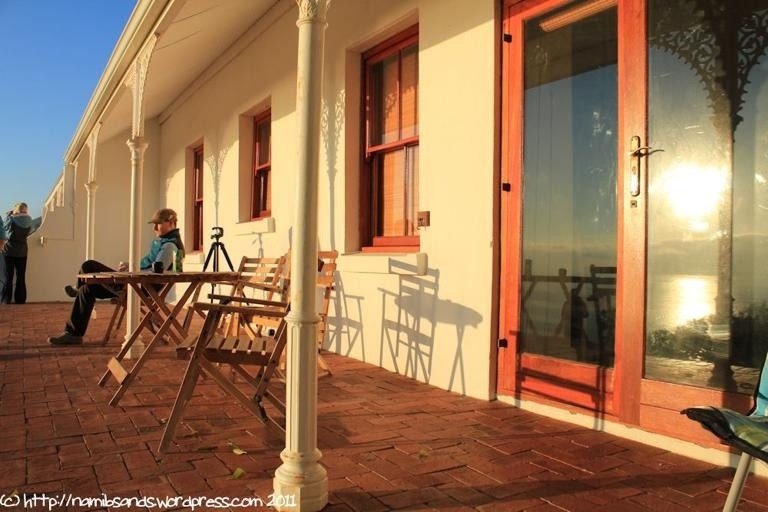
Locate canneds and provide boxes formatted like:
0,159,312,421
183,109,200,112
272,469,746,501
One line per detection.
172,249,183,273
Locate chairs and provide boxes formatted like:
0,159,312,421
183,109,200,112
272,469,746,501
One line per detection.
98,277,177,347
157,251,336,452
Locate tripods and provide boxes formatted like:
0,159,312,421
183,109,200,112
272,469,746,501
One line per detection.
202,227,251,306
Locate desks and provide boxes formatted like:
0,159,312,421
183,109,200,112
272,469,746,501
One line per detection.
77,270,240,406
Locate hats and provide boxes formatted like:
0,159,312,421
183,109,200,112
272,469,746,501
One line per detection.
147,208,177,224
16,203,28,212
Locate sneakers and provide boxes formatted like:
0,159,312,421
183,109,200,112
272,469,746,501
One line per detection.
48,332,83,345
65,285,77,298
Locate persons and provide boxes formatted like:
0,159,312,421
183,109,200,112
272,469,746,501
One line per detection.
47,208,186,345
0,202,33,305
560,288,589,340
